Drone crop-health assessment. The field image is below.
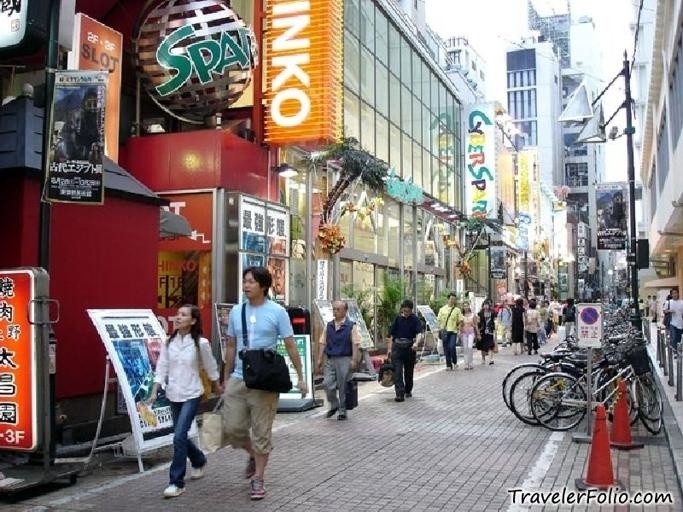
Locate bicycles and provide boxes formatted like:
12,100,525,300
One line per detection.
502,303,665,437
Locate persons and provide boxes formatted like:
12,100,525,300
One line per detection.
538,300,547,330
663,288,683,358
146,304,223,497
562,297,576,337
510,299,525,355
645,295,651,317
638,299,645,316
222,266,310,498
496,303,512,347
549,297,561,333
544,300,551,338
317,300,362,420
477,301,494,364
558,301,563,325
437,292,461,371
650,295,657,322
525,301,540,355
387,300,423,401
459,301,481,369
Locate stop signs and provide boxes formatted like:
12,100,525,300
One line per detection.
577,303,602,350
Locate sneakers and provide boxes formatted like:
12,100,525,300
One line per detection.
445,367,452,372
244,454,257,478
249,476,267,499
191,460,209,480
453,364,460,371
406,391,413,398
394,393,405,402
163,484,186,498
337,413,348,421
326,408,338,417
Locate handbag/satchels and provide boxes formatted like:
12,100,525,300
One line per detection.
378,359,395,387
239,304,293,393
393,338,412,351
493,329,497,353
439,306,455,341
201,397,229,456
345,378,358,410
663,301,673,329
